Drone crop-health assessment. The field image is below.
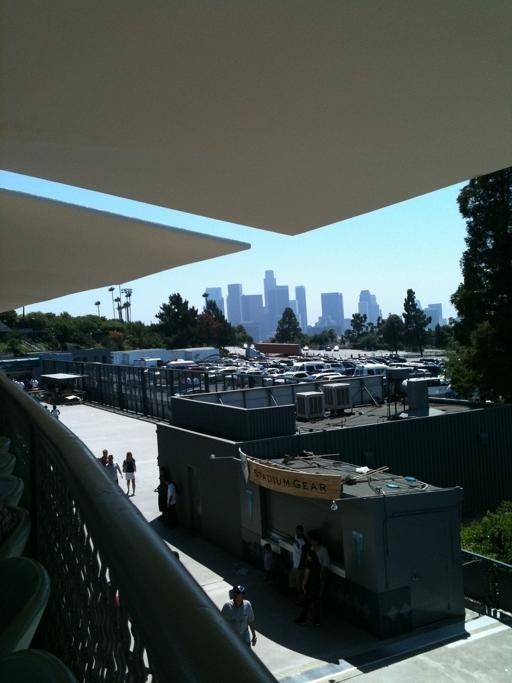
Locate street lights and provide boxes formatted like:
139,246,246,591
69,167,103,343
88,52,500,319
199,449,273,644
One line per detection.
202,292,209,307
94,286,132,323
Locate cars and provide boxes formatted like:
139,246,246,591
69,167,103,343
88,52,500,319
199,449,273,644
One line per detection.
130,352,452,401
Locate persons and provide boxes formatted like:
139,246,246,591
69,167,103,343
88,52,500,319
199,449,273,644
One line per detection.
99,449,111,469
290,524,330,627
122,452,136,496
220,585,257,648
10,376,39,390
44,405,49,413
105,454,123,486
50,404,60,420
164,478,177,530
262,543,277,582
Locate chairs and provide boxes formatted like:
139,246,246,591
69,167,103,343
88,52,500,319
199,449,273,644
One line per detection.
0,436,11,453
0,452,16,476
0,648,81,683
0,474,25,506
0,557,52,657
0,504,32,559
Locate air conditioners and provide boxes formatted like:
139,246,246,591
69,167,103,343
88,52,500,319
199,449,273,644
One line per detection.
296,391,324,420
323,383,352,410
